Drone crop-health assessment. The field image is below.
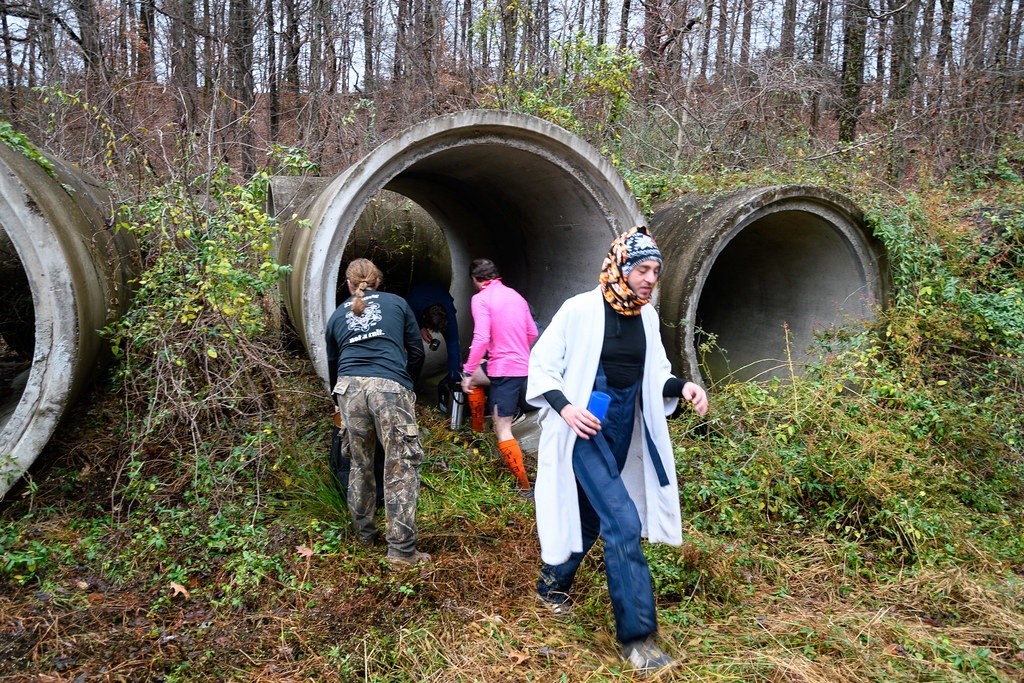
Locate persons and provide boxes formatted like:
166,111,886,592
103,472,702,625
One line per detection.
405,285,462,389
526,224,709,670
325,259,433,566
460,257,538,499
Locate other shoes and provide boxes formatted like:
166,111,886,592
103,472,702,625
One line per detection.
387,550,432,571
622,638,681,680
520,489,534,499
534,595,570,617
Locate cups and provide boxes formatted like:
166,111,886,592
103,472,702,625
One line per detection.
429,339,440,351
588,392,611,425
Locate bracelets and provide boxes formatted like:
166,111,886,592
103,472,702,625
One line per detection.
462,372,471,377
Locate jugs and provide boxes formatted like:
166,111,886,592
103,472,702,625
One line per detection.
450,381,465,430
